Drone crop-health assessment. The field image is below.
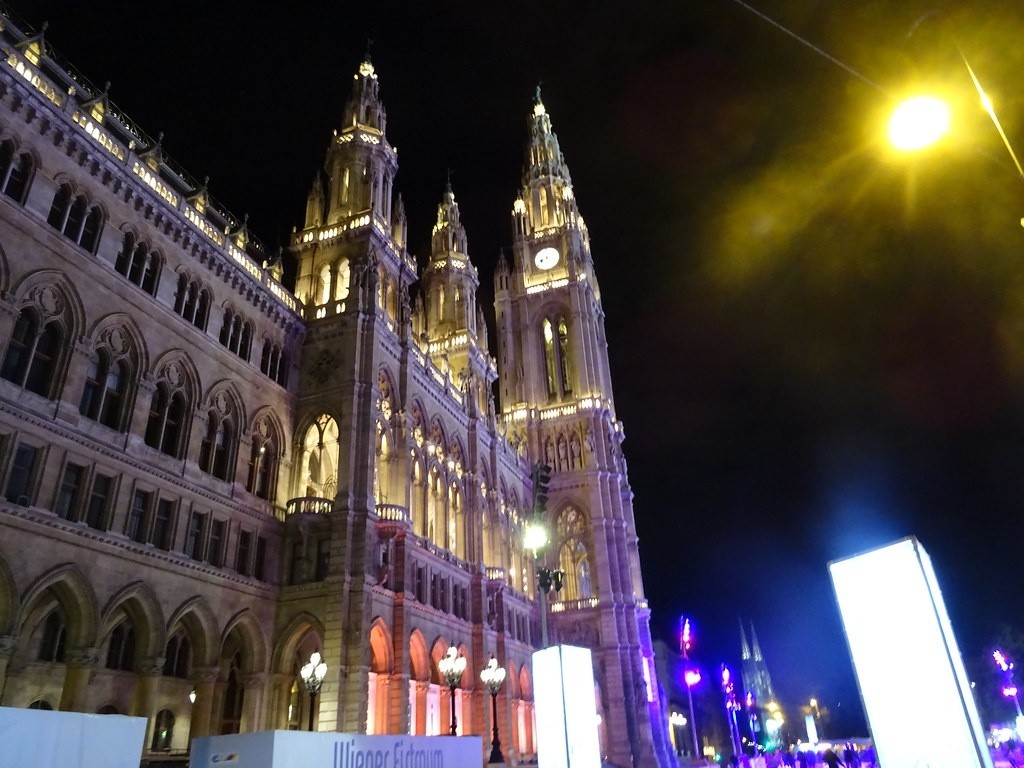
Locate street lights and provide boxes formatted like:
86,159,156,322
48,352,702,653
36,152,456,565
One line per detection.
299,650,327,731
479,656,508,764
887,10,1024,181
532,462,551,649
671,712,689,757
438,643,466,737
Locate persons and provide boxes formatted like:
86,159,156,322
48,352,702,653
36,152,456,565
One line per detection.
717,736,1024,768
